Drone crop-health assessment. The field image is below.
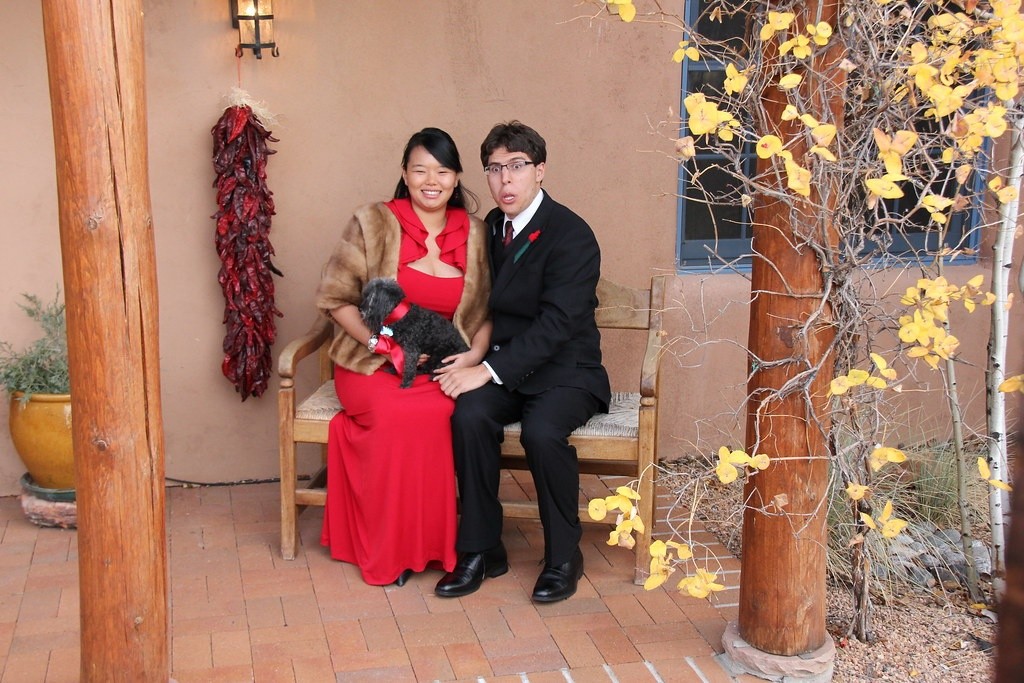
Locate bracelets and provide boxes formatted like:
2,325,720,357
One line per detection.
368,334,378,353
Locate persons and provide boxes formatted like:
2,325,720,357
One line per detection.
432,120,611,602
315,127,492,587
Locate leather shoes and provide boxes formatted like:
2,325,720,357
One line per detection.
434,540,507,596
532,546,583,601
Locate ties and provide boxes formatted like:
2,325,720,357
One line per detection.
502,221,514,249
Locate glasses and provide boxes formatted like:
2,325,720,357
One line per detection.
485,160,534,175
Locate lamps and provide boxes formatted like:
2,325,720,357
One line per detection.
230,0,280,60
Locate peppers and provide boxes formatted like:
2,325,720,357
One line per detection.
210,103,285,404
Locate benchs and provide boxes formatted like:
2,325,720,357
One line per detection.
273,267,668,585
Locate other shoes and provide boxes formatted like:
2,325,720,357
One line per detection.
395,569,411,586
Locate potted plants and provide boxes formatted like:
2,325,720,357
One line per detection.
0,284,75,490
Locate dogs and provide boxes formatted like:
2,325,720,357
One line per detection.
359,277,471,389
